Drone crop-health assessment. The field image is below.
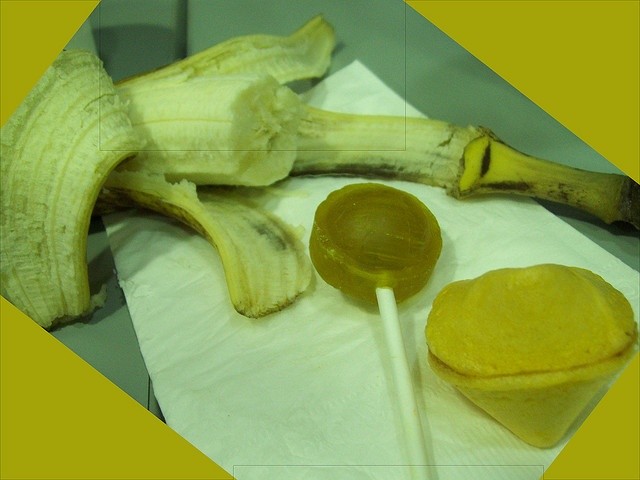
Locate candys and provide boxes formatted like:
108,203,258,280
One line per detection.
309,182,442,478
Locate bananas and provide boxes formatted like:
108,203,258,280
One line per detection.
0,15,639,329
